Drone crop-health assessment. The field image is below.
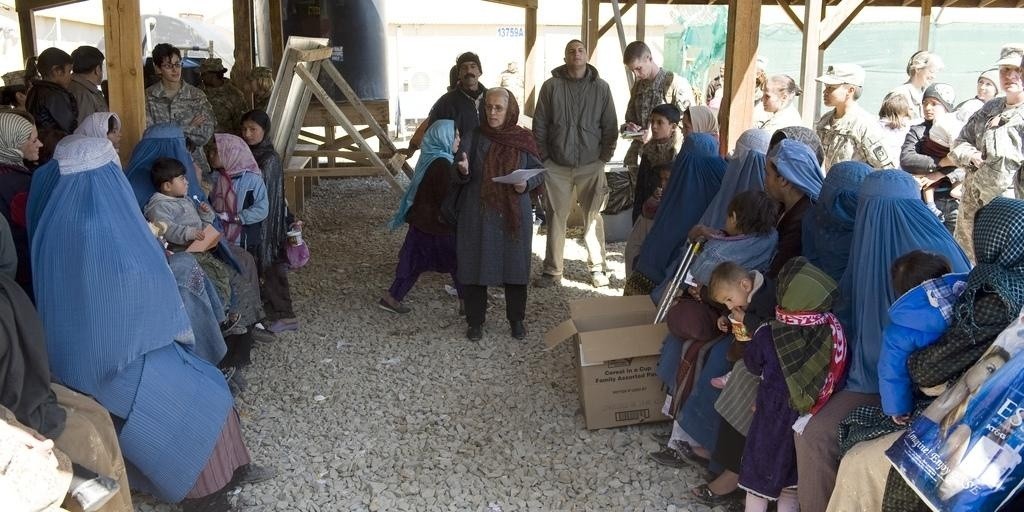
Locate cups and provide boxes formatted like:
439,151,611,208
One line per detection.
729,315,752,342
288,232,302,247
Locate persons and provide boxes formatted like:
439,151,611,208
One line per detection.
425,51,489,155
619,37,1022,512
376,117,468,318
0,38,310,510
530,38,619,290
445,85,546,343
393,66,460,160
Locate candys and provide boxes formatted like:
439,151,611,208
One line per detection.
193,194,203,204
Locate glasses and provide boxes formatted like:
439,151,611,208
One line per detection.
486,105,508,112
161,62,182,69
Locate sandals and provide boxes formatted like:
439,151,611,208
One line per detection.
218,311,241,334
221,365,236,382
690,484,746,504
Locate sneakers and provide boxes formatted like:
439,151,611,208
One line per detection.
246,324,277,342
534,273,563,288
673,439,709,472
459,303,494,319
233,463,278,484
590,272,610,287
649,430,672,445
509,320,526,340
377,297,411,314
648,448,690,468
267,319,298,333
467,323,482,341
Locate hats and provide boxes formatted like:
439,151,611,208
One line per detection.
246,67,272,81
909,51,930,70
977,68,1002,95
194,58,228,73
814,62,865,87
995,42,1023,68
922,83,956,112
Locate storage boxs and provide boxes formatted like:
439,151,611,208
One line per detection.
542,288,682,431
